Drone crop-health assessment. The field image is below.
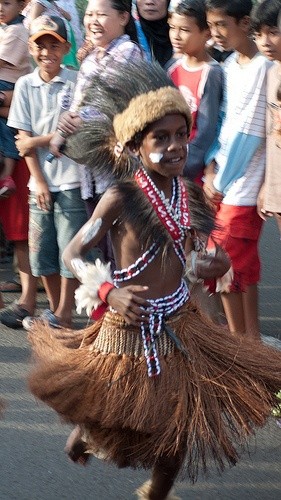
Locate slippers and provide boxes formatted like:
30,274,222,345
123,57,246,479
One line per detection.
0,298,60,334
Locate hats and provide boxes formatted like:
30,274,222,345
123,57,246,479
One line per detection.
63,42,192,180
27,14,68,44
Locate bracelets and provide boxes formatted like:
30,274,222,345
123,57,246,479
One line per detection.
90,280,115,323
0,91,6,106
57,129,67,137
0,0,281,500
